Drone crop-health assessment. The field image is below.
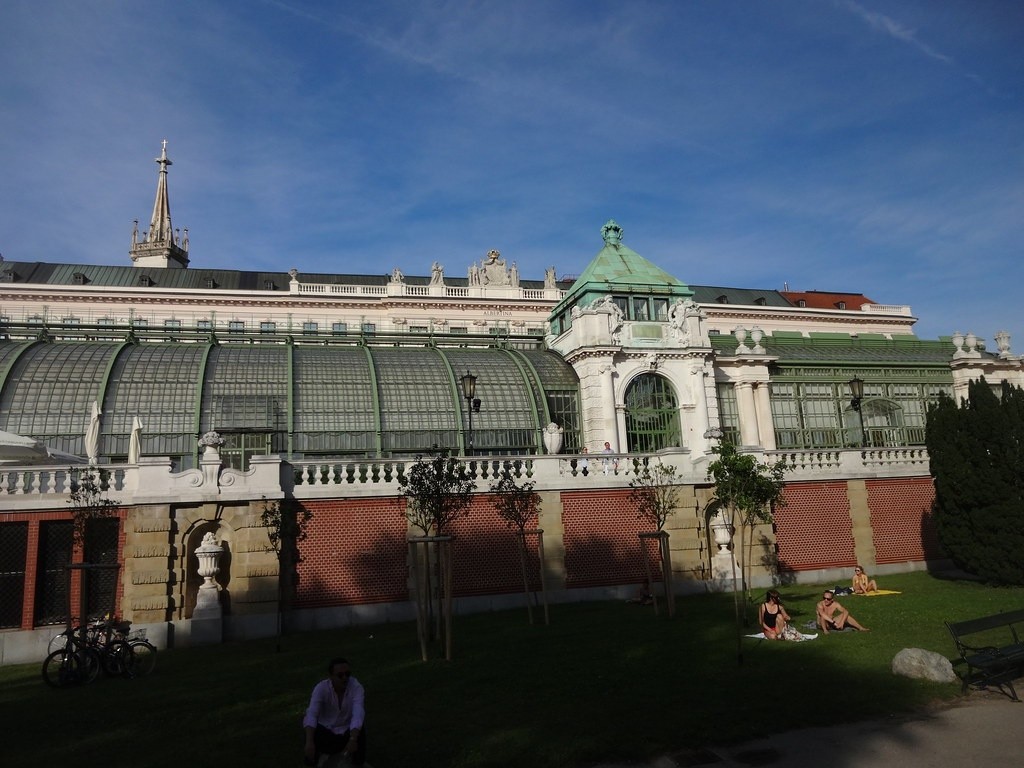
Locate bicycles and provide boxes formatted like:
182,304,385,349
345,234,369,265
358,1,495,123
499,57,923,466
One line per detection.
42,615,160,691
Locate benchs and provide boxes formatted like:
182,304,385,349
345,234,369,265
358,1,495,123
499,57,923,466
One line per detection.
945,609,1024,702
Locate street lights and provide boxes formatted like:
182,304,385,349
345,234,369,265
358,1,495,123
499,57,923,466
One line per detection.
458,371,481,456
847,374,868,447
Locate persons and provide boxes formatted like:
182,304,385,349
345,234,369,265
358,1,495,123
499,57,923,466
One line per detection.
815,589,870,635
758,589,791,640
391,267,404,283
302,657,367,768
852,566,879,595
431,261,444,285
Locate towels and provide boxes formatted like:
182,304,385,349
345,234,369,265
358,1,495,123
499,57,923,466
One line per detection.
827,586,902,597
800,618,862,634
744,620,819,643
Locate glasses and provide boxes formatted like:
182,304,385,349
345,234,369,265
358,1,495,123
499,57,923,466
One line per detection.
855,570,859,572
823,597,831,601
336,671,351,678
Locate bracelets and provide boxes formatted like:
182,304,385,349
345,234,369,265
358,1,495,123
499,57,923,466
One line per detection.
350,737,355,741
832,621,835,625
